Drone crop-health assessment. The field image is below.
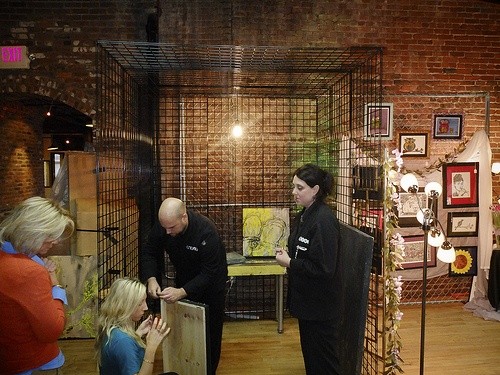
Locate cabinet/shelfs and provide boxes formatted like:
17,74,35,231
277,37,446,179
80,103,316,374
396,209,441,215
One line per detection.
62,149,139,299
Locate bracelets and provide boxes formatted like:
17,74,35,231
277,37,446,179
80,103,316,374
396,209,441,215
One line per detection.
143,359,154,364
52,284,64,289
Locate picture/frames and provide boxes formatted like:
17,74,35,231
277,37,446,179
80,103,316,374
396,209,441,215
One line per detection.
446,212,479,238
389,234,436,271
395,130,430,159
442,162,479,209
361,209,383,230
432,112,464,142
363,102,394,141
392,187,438,228
448,246,478,277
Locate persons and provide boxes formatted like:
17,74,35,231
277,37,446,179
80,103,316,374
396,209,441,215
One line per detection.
275,163,346,375
0,194,74,375
142,197,228,375
94,278,179,375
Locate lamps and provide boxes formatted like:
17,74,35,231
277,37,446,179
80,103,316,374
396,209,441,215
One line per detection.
492,162,500,176
0,46,35,69
402,174,458,375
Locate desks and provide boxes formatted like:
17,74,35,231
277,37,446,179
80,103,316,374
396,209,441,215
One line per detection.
227,262,287,333
